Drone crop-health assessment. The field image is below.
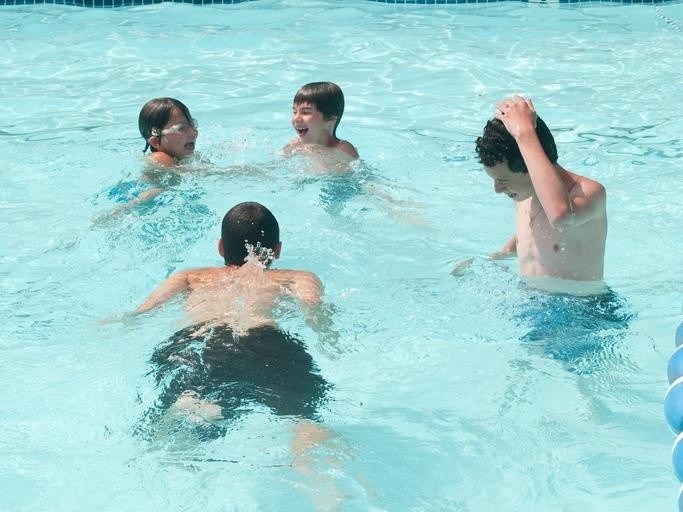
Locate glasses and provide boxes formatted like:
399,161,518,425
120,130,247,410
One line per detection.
151,119,198,136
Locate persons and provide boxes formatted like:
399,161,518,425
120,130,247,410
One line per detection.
444,95,608,296
132,97,200,186
101,201,343,512
276,78,358,181
